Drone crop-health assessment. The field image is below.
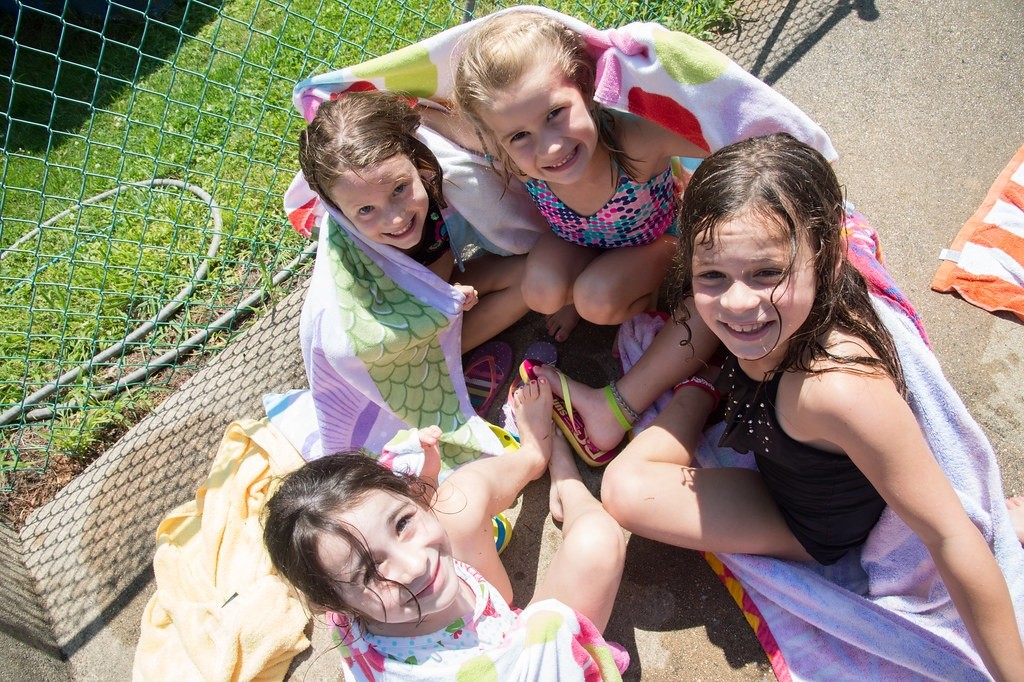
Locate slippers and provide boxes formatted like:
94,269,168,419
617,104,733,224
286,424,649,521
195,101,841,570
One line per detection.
520,359,622,469
463,337,515,418
505,337,559,420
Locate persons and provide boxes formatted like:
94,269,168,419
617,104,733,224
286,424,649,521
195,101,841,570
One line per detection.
260,376,630,682
296,91,531,357
517,130,1024,682
414,10,713,358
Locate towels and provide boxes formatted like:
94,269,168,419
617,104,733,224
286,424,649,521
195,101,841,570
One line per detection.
132,5,1024,682
